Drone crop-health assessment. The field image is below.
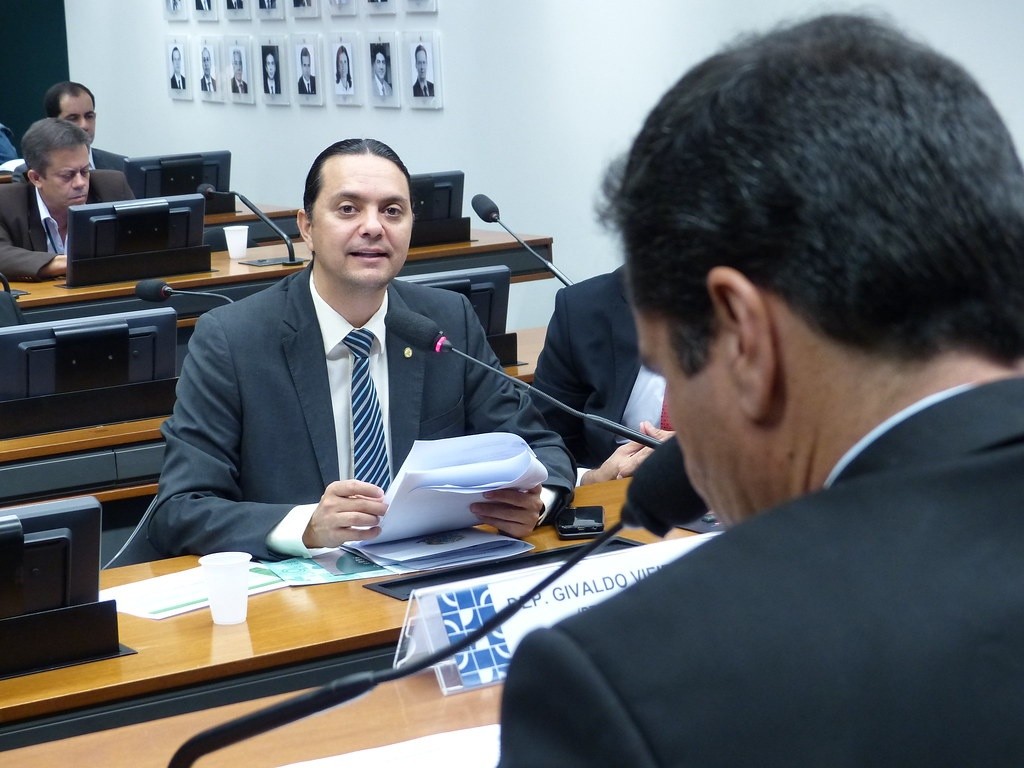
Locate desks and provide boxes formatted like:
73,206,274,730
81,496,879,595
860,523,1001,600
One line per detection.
1,204,703,723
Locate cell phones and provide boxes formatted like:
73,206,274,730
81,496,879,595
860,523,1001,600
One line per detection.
555,506,605,539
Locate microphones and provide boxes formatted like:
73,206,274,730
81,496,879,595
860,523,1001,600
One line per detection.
196,184,301,266
471,194,574,288
383,307,661,450
133,280,234,306
168,436,712,768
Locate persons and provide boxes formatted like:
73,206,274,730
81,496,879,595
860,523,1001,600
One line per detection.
0,123,19,165
334,46,352,93
171,0,388,10
170,48,186,89
413,45,434,97
298,47,316,94
263,54,281,94
498,13,1024,768
0,117,138,283
201,47,216,91
148,139,577,562
529,264,676,487
12,81,141,198
371,45,392,95
230,49,247,93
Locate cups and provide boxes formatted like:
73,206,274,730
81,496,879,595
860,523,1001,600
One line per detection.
198,552,252,625
223,226,249,259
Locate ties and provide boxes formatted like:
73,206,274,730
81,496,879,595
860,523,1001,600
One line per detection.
661,384,674,433
343,329,391,500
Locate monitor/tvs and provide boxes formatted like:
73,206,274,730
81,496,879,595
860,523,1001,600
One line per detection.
0,148,505,620
